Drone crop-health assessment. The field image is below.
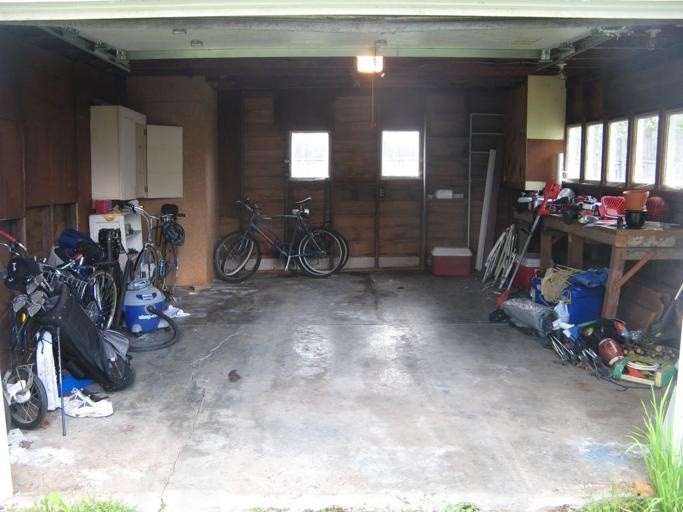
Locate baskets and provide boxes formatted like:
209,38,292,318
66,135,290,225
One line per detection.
598,196,626,219
41,265,88,299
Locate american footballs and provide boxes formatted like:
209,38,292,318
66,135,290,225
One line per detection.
598,337,624,367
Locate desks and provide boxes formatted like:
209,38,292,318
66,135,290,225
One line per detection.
512,207,683,318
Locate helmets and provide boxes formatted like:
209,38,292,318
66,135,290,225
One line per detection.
162,221,185,245
98,228,120,248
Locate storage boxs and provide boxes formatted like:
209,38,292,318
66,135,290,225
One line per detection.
514,252,553,290
534,278,605,326
430,247,473,277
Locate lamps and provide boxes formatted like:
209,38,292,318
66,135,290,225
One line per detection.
534,25,628,71
39,26,131,73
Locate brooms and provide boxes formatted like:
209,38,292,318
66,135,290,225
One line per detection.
497,198,548,309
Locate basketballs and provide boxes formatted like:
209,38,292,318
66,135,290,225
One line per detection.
645,196,671,222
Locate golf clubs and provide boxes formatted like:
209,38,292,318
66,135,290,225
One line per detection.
11,273,56,317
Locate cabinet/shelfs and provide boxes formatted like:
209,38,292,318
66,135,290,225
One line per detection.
91,106,183,200
89,213,143,283
503,79,564,192
462,113,505,249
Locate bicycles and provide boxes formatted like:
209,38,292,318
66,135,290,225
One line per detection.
213,196,349,284
116,202,185,293
0,229,119,430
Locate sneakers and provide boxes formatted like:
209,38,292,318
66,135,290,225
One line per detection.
59,388,113,418
